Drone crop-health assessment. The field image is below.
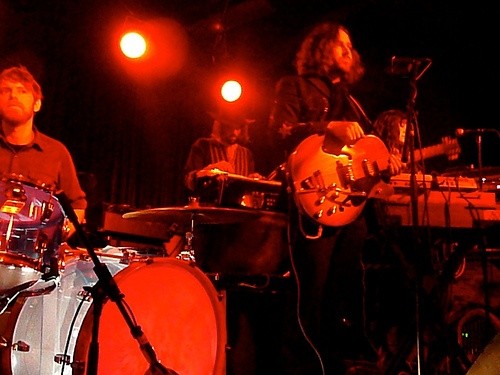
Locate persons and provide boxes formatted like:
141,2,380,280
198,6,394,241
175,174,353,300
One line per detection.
181,110,264,209
0,66,87,239
268,23,412,374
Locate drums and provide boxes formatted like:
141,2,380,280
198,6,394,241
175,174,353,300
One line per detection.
99,202,171,240
0,175,65,298
0,249,227,375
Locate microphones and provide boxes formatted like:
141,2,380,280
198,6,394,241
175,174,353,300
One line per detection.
456,128,496,137
385,56,423,74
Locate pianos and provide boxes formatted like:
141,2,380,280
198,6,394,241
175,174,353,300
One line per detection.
195,167,284,203
389,175,478,227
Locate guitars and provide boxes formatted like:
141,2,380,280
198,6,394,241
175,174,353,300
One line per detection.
287,130,464,227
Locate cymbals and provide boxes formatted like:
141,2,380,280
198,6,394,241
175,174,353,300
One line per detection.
120,205,262,224
